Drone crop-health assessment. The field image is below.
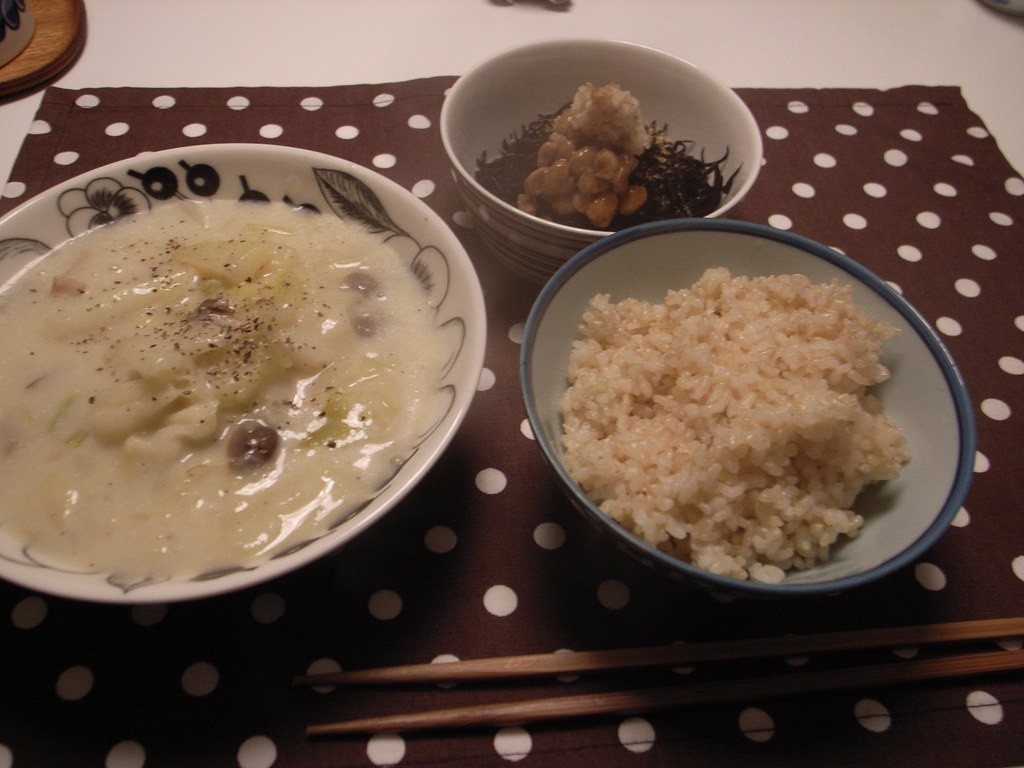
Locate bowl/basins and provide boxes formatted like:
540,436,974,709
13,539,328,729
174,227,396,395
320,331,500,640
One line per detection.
519,218,976,594
1,141,489,606
439,37,766,291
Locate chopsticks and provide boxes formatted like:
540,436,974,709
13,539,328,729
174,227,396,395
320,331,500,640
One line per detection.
294,615,1023,734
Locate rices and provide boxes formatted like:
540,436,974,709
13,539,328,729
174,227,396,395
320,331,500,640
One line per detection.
557,267,914,584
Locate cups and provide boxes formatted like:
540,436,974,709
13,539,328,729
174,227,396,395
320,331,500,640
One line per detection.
0,0,36,67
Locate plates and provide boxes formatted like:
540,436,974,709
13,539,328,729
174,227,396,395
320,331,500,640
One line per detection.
0,0,87,95
